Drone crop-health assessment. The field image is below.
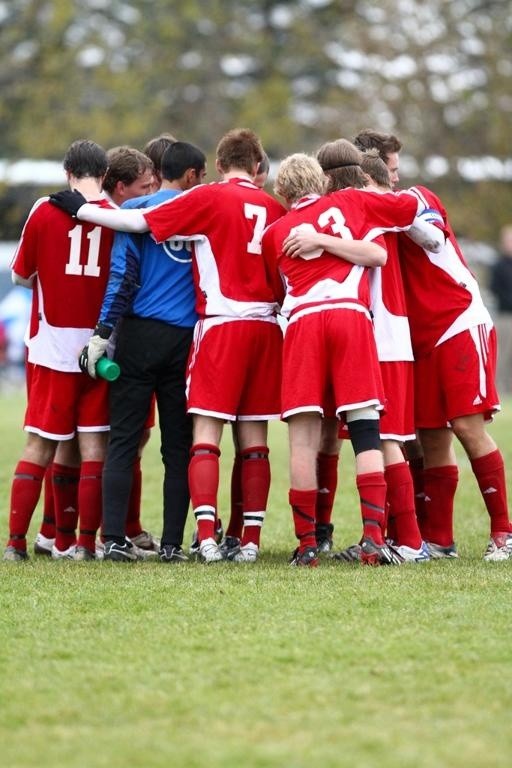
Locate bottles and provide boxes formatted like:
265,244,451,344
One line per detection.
75,345,123,383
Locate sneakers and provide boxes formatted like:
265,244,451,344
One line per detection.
315,523,334,555
289,547,322,568
331,536,459,568
483,524,512,564
3,531,160,564
158,525,259,564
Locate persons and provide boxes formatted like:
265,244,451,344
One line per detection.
259,129,511,569
3,128,287,568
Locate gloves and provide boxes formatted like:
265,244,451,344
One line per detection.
49,189,87,221
82,321,113,381
